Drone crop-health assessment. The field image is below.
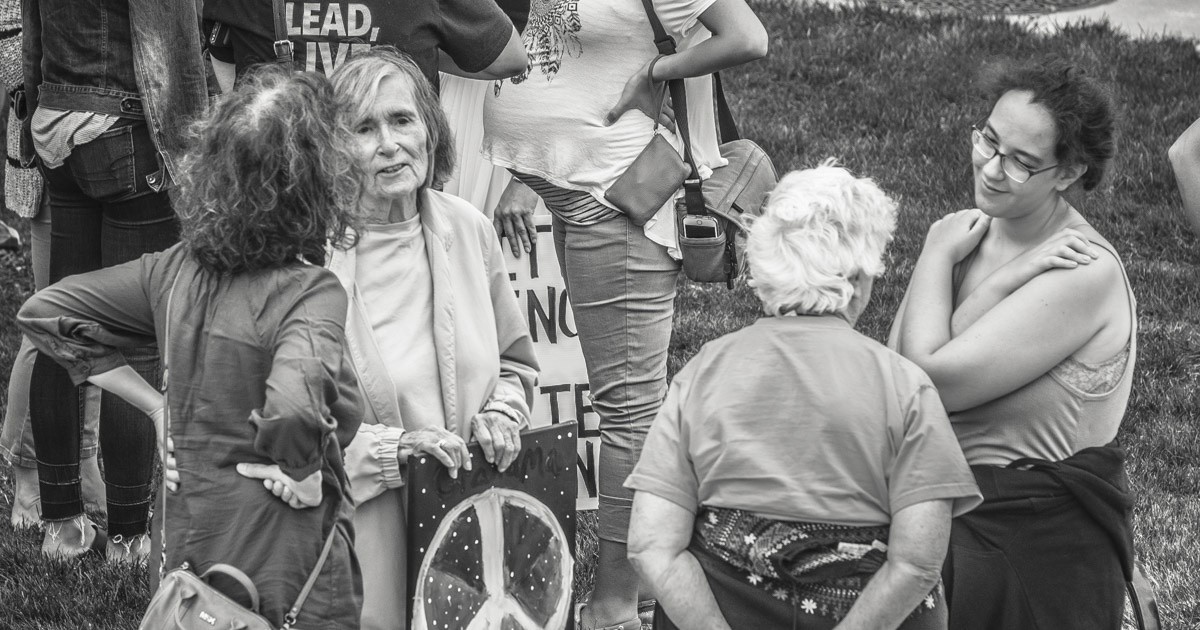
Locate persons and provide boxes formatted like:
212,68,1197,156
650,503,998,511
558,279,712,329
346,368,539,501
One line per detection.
622,157,984,630
0,0,539,553
296,45,542,630
483,0,768,630
12,64,367,630
886,62,1137,630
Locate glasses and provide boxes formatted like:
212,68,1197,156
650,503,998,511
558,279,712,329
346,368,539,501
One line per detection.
971,112,1072,183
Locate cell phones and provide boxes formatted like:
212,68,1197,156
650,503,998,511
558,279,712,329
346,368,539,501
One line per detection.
681,214,718,238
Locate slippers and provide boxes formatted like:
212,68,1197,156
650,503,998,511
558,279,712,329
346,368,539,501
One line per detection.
83,525,108,561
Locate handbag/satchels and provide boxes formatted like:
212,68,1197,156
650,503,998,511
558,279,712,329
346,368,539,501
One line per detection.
137,563,277,630
675,138,779,282
603,133,690,225
